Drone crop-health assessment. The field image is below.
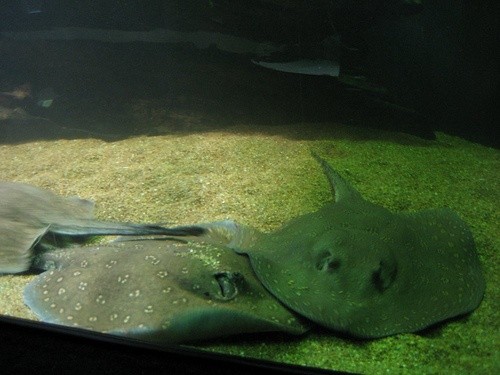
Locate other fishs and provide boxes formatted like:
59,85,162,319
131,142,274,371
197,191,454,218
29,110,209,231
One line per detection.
0,180,206,275
22,218,312,347
249,55,341,77
206,150,488,337
